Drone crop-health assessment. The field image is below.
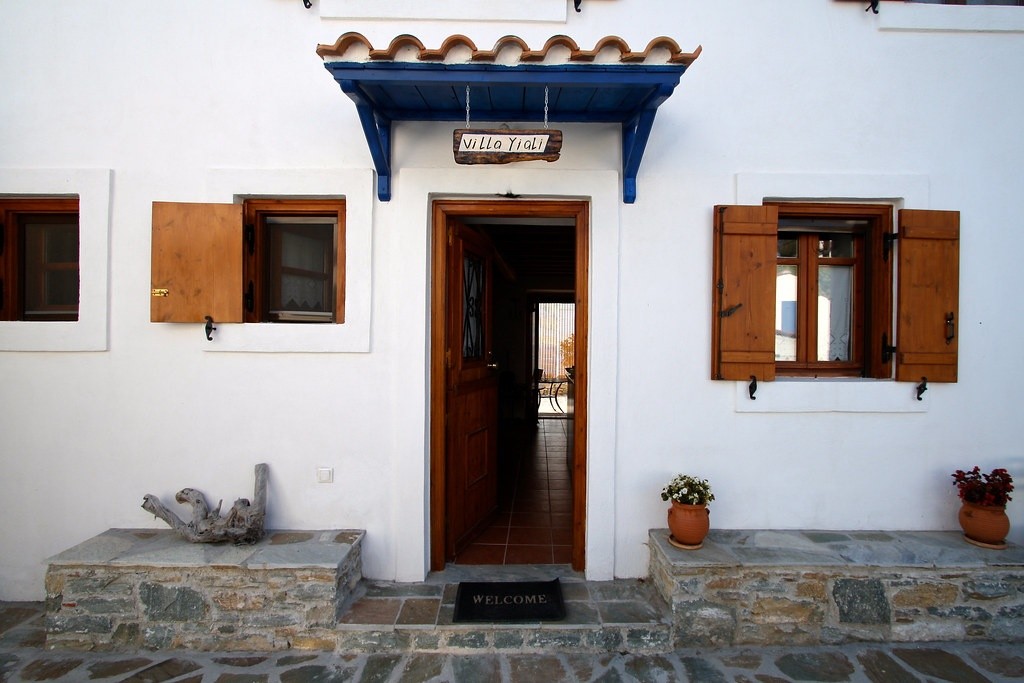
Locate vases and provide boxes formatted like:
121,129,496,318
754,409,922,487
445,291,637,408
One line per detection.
959,499,1010,550
668,499,711,550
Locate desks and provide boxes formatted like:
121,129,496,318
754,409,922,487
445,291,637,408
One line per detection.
537,378,570,414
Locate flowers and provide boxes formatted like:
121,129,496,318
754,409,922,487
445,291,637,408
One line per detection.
661,473,716,504
951,466,1015,509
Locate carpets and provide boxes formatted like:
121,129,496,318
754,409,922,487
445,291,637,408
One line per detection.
450,577,568,624
